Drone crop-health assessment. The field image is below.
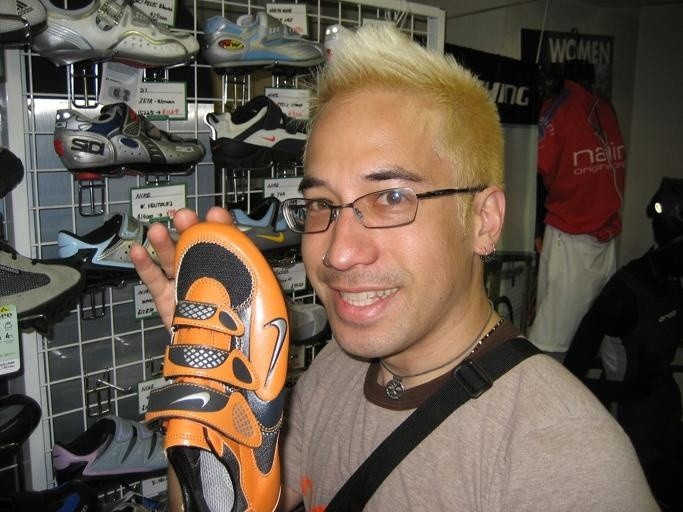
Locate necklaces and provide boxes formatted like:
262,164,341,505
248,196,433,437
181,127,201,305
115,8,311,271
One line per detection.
377,297,495,400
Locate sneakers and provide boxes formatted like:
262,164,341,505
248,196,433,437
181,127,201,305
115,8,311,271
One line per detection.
0,481,101,512
1,394,41,466
226,197,305,251
60,211,180,292
206,95,311,168
55,103,206,171
0,1,199,69
1,240,85,329
146,223,289,512
52,416,169,488
205,11,325,67
0,147,24,199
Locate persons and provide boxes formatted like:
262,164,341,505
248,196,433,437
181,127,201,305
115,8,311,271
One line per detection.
129,24,662,512
528,58,632,357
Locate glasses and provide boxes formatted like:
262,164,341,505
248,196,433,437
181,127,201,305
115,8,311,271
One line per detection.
282,187,489,234
